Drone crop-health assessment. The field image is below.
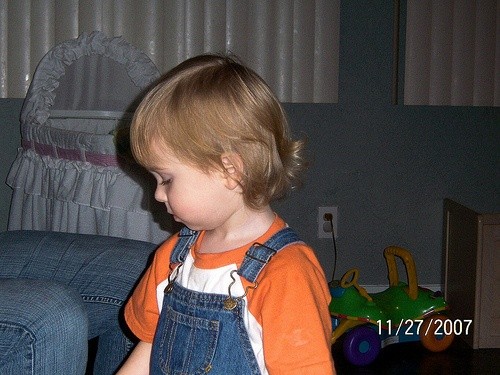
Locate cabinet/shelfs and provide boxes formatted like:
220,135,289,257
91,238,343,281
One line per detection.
443,196,500,349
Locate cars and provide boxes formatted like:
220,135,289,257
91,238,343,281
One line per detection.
328,246,455,368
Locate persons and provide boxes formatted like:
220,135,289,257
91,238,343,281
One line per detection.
1,229,161,375
113,53,337,375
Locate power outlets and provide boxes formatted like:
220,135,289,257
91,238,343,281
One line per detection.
318,206,338,239
